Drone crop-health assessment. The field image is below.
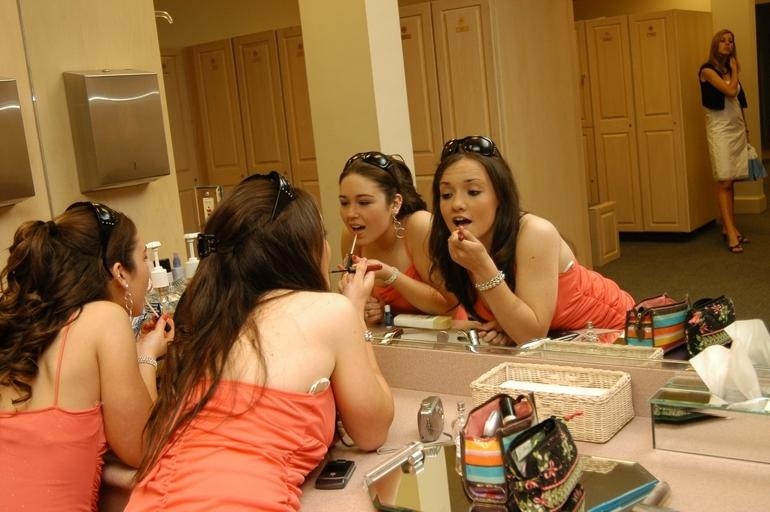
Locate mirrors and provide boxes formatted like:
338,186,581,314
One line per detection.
17,1,717,345
369,440,658,512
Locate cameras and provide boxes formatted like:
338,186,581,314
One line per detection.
418,395,445,443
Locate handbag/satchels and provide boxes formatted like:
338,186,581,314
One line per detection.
503,415,585,512
625,294,692,357
458,391,540,505
684,294,737,357
748,143,768,181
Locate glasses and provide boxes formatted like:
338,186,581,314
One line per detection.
441,135,504,165
344,152,401,193
65,201,114,279
239,170,297,221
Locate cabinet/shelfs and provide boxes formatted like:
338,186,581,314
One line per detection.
574,1,720,234
160,2,598,270
587,202,622,269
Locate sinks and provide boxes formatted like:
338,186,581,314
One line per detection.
401,331,487,345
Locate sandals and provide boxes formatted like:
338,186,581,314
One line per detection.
722,233,751,243
728,243,744,253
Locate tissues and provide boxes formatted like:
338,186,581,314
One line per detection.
649,344,770,465
725,319,770,376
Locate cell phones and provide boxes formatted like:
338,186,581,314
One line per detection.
547,330,580,343
315,459,357,490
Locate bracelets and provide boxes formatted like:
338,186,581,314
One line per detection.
363,330,373,341
384,266,399,284
474,270,506,293
137,356,157,371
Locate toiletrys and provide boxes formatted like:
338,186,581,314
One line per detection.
145,240,182,319
393,314,454,330
499,397,517,424
169,232,201,295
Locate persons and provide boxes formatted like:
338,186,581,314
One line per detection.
122,171,394,512
422,136,636,346
338,151,469,322
698,29,752,253
0,201,177,512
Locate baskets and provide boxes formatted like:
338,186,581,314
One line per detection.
469,361,634,444
516,339,664,370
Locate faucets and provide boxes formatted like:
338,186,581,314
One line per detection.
379,327,403,344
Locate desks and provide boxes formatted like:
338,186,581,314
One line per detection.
366,316,625,355
102,347,770,512
